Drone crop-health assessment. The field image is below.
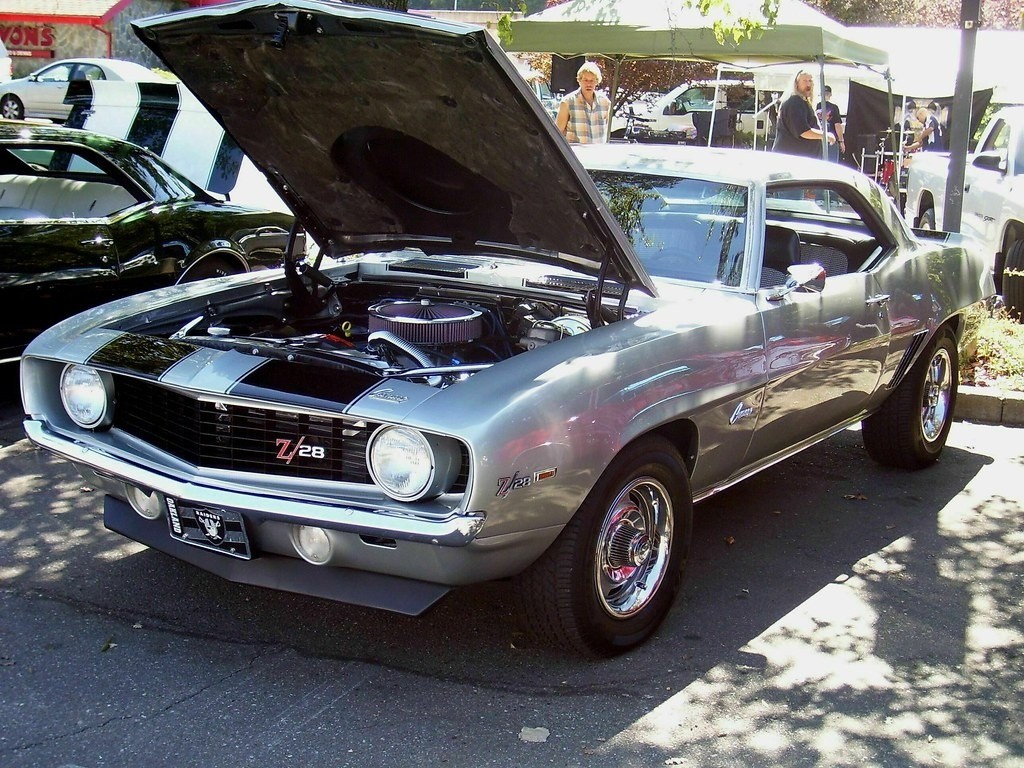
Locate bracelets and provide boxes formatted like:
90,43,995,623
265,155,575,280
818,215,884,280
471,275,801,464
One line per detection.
838,140,843,144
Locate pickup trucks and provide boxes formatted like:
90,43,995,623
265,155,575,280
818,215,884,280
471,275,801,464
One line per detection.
900,96,1024,325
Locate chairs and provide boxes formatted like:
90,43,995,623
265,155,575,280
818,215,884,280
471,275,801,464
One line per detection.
729,224,801,290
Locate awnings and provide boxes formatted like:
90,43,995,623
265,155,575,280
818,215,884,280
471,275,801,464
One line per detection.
0,0,128,25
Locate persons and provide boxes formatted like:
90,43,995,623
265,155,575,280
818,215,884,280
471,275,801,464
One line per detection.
555,62,611,143
904,102,945,152
771,70,835,200
816,85,845,163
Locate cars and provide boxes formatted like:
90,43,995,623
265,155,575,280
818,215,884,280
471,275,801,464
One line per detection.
0,121,309,384
1,57,166,127
16,2,979,664
521,68,669,136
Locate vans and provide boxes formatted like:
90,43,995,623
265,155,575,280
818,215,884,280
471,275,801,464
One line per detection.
627,78,778,146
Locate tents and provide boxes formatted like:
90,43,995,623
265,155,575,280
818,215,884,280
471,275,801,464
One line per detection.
499,1,900,213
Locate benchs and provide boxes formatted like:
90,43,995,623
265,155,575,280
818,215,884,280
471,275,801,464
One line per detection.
704,222,860,277
0,174,138,220
